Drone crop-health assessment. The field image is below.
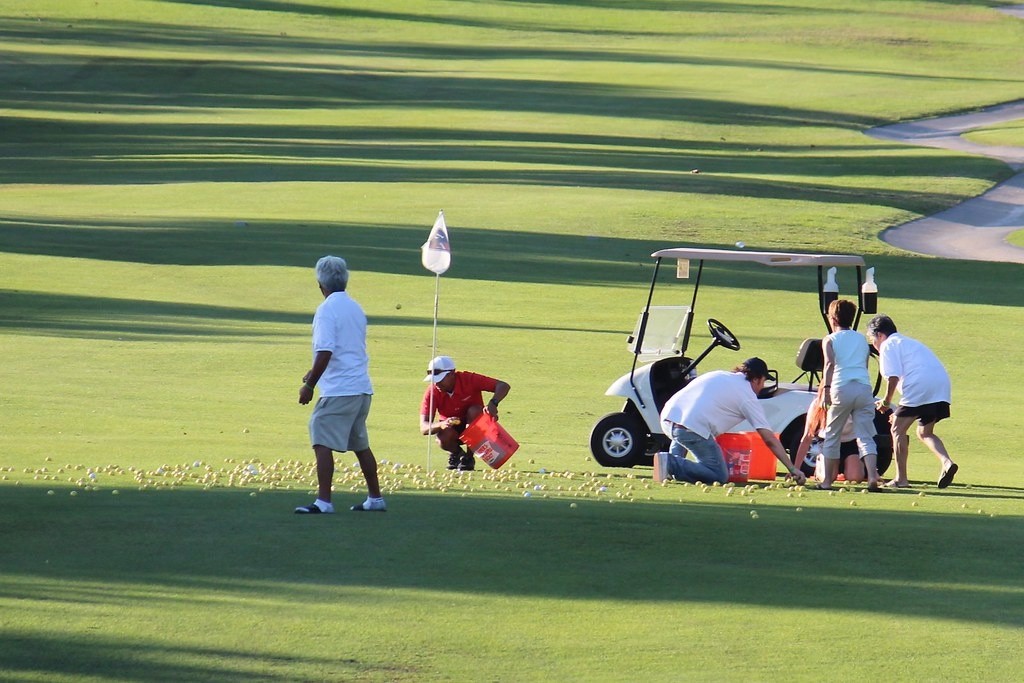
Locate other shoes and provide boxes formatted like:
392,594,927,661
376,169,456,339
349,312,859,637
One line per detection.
351,499,387,511
447,447,465,469
295,504,334,514
654,452,668,482
457,453,475,468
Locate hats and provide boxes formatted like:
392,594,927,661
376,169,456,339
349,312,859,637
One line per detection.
744,357,776,381
424,356,455,382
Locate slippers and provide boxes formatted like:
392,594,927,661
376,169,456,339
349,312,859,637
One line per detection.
809,484,838,490
868,488,882,492
880,479,912,488
938,464,958,488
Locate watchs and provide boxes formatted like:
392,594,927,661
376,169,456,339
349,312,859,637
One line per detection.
490,399,498,406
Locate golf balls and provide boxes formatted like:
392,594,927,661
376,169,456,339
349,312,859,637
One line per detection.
395,304,403,309
70,491,78,497
978,508,985,516
964,484,973,489
112,490,121,496
15,480,22,487
44,475,50,480
243,429,251,434
600,487,606,492
0,455,129,493
128,457,478,497
251,470,259,474
89,473,96,478
2,475,10,481
991,512,1000,521
524,493,531,499
661,477,941,519
248,465,254,470
193,462,200,467
480,456,657,510
157,468,165,472
52,476,59,481
534,485,540,490
47,490,55,496
962,504,970,511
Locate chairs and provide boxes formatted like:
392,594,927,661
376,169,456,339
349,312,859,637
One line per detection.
791,338,824,392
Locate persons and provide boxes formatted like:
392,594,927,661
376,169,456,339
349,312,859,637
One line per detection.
867,313,958,489
653,357,807,486
420,356,511,471
804,300,883,492
294,256,387,513
786,378,864,484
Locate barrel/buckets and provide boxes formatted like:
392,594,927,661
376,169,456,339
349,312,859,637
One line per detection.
714,429,751,482
742,431,783,481
458,410,520,471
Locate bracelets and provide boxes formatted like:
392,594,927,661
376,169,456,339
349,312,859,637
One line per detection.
305,383,314,390
823,385,831,388
882,399,890,407
789,466,796,472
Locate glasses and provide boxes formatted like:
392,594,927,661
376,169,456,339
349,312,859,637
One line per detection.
427,369,452,375
